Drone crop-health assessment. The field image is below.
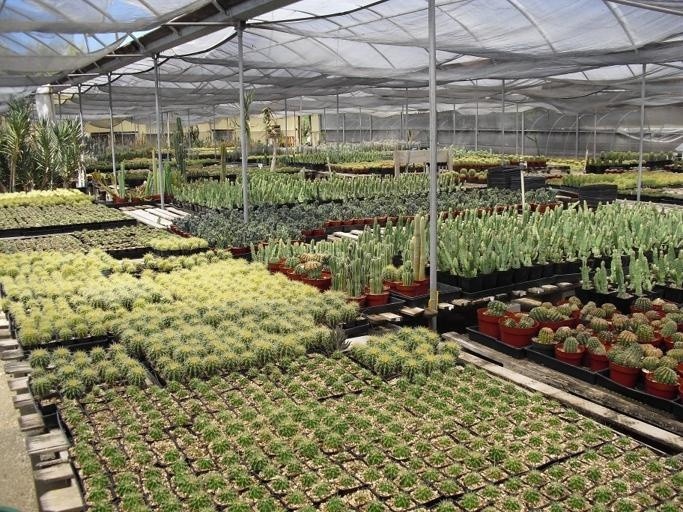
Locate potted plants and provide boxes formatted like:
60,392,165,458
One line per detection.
0,154,682,512
